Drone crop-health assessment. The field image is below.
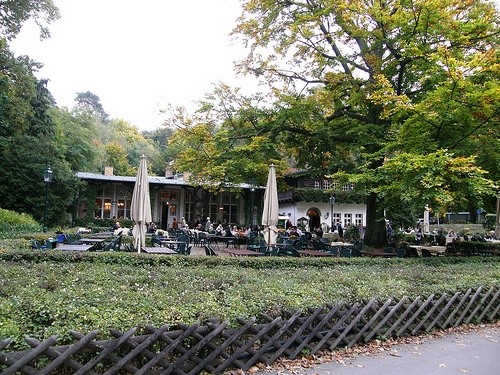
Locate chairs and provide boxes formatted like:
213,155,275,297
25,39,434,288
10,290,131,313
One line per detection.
384,235,472,258
35,233,82,249
151,228,363,258
102,229,134,251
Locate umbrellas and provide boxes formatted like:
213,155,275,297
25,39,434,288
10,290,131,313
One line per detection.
130,153,152,254
262,163,278,249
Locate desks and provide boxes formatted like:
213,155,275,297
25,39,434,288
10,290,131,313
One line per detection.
55,232,114,251
219,237,236,248
298,249,332,258
159,238,174,246
161,241,185,249
410,246,447,256
272,244,292,255
141,247,178,253
224,250,265,256
446,236,472,244
325,243,354,250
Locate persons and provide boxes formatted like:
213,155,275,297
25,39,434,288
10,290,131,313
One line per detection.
473,230,498,242
113,216,120,220
173,216,250,238
148,221,159,233
123,222,127,235
386,220,456,240
251,220,365,250
